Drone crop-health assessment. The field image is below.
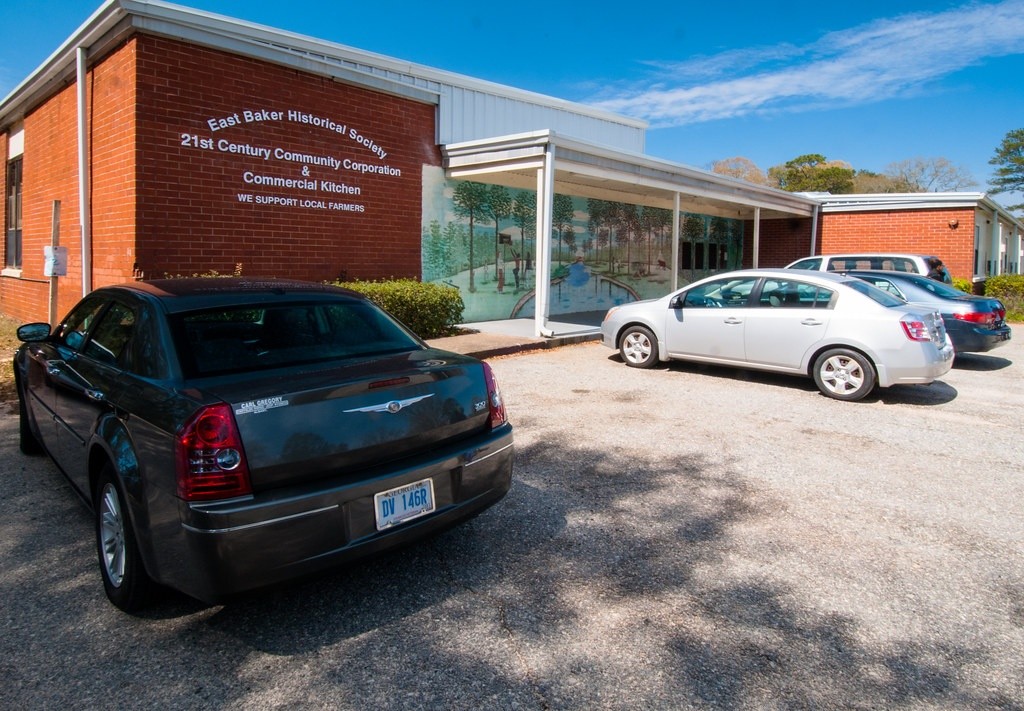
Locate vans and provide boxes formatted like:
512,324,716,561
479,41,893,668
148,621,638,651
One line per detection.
730,253,952,296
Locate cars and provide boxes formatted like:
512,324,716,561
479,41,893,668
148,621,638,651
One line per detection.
11,278,516,618
770,269,1011,362
599,266,957,402
